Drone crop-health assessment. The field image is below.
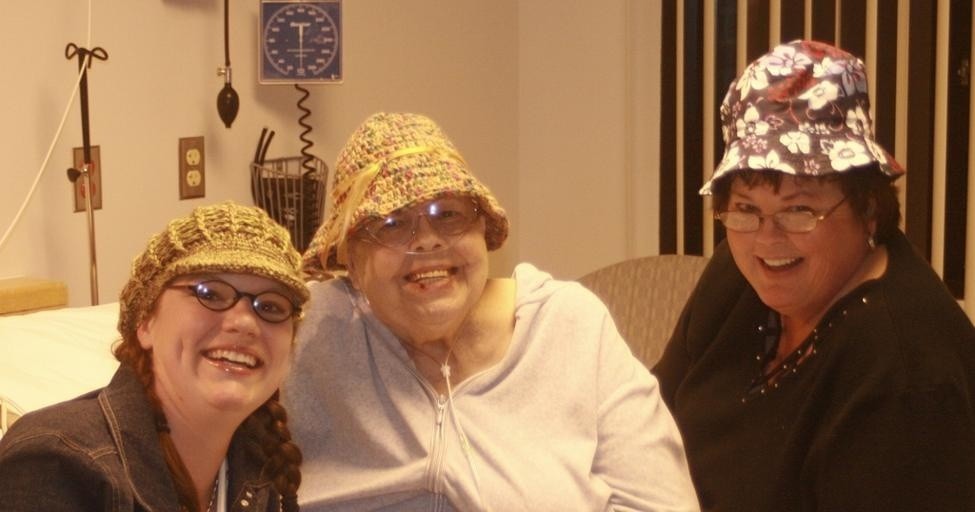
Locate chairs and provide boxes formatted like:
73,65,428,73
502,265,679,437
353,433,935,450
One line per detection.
577,254,710,371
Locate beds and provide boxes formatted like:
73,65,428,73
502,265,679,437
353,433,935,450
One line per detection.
0,274,437,512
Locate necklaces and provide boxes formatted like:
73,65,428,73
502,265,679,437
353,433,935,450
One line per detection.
200,479,221,512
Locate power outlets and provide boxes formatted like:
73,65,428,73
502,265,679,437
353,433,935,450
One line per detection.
178,135,206,201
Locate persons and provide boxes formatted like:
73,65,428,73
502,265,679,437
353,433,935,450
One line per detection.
0,195,311,512
640,39,975,512
271,109,702,512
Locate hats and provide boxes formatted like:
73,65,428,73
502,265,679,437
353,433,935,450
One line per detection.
117,200,310,339
301,113,509,271
698,40,904,195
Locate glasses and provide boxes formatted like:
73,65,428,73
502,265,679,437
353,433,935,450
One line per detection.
347,198,484,246
714,196,848,233
167,280,301,323
